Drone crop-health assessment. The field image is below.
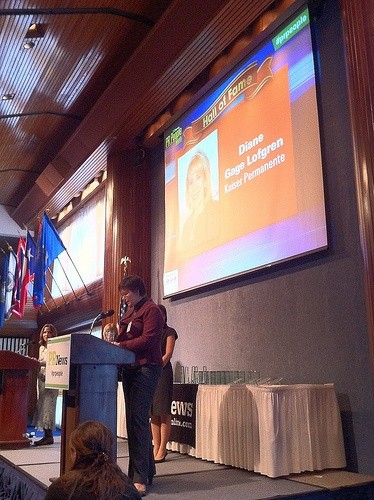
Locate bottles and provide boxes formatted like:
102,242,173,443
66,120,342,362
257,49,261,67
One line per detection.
180,366,207,384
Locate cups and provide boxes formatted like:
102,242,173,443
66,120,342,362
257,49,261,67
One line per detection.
195,371,260,384
108,330,115,344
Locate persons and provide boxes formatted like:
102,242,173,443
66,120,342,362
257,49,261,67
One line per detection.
44,420,144,500
107,274,162,495
153,304,178,463
24,323,59,445
181,150,218,236
102,324,118,343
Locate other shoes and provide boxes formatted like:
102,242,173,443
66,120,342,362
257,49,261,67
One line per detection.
133,482,147,497
34,437,55,446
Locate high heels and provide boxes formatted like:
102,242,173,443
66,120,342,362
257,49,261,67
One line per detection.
153,450,167,463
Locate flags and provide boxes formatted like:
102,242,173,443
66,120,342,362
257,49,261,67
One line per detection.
0,210,66,328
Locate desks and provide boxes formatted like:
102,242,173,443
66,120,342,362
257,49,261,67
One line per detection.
117,381,347,478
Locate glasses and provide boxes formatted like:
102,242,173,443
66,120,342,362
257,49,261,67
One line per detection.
121,291,136,298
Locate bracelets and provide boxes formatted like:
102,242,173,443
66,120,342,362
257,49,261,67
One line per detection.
112,340,116,345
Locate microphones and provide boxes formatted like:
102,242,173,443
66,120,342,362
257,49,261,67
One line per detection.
20,341,36,346
89,310,114,334
